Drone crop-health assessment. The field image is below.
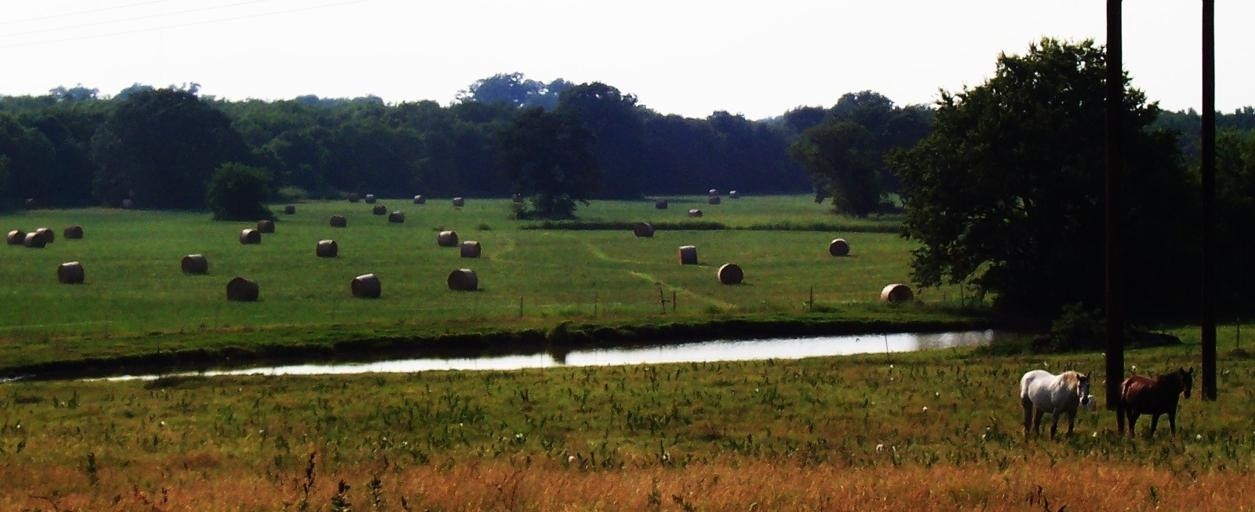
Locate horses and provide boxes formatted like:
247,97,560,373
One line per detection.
1117,367,1195,439
1018,369,1091,444
